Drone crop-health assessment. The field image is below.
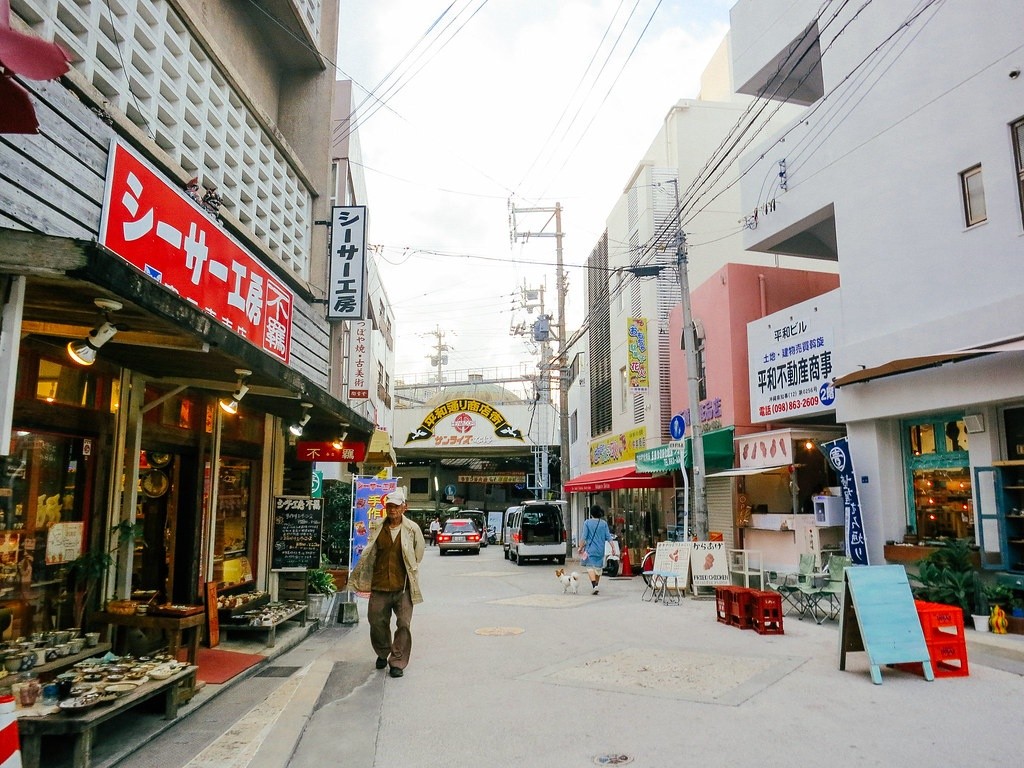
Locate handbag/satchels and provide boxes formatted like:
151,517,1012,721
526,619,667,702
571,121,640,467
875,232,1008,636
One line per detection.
579,550,587,561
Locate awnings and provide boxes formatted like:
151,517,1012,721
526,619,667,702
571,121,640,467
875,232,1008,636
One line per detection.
564,467,673,492
0,227,375,443
340,428,397,466
635,429,733,472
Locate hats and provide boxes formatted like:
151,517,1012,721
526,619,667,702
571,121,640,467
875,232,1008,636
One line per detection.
386,491,405,505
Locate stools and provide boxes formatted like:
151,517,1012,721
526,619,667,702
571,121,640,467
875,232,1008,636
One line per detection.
642,571,682,606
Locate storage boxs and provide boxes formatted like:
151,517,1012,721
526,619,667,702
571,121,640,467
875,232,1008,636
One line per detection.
714,586,784,635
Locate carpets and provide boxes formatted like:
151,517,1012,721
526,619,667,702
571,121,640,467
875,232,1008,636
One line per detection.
178,643,270,684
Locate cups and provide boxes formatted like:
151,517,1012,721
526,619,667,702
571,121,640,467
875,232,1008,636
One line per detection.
0,627,100,706
136,605,149,616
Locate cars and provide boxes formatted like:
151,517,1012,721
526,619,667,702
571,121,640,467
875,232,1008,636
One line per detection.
438,518,481,556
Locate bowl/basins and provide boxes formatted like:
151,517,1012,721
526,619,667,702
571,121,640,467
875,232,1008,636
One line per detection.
56,655,191,715
110,600,137,615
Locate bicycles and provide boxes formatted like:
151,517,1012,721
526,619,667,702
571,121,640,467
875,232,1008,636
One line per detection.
641,528,697,590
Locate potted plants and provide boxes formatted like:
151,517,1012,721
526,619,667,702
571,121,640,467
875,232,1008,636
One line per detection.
306,568,337,619
908,537,1024,635
322,564,348,591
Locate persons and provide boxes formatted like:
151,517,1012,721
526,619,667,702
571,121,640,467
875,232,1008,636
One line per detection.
347,492,425,677
577,505,616,594
441,517,450,533
430,517,440,546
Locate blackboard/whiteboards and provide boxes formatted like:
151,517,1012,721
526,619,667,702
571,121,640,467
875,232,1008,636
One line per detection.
271,495,324,571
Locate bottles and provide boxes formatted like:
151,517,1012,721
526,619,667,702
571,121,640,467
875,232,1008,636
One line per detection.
0,695,17,714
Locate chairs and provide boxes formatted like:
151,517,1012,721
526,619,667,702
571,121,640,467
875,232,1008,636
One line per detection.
534,516,552,536
766,553,852,624
522,518,531,533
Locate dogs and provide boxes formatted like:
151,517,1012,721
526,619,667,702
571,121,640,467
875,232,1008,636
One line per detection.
555,569,580,594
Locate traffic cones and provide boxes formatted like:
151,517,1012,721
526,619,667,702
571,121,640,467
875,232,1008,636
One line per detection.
622,544,633,576
644,545,653,571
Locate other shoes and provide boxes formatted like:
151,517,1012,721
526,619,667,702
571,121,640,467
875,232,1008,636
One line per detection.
376,657,387,669
592,586,600,595
389,663,403,677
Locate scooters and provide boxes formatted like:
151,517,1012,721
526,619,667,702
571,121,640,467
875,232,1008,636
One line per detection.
479,526,497,544
602,528,626,577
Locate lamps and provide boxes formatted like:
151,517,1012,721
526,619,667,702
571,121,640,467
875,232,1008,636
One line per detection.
66,297,122,366
220,369,252,415
333,423,349,449
290,403,313,436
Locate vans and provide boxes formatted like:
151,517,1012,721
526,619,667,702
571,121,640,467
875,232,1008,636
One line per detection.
458,510,487,547
503,500,569,566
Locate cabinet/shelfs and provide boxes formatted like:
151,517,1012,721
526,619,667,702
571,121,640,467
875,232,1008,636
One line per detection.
727,549,764,591
